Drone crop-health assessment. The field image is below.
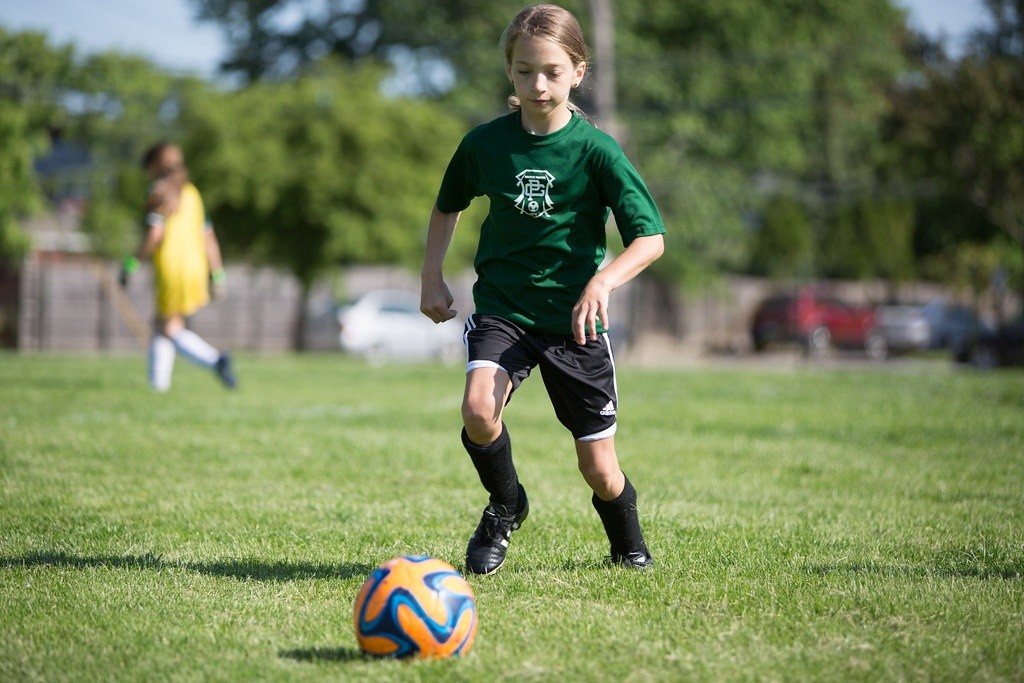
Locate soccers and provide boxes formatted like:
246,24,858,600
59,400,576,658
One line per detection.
353,554,477,661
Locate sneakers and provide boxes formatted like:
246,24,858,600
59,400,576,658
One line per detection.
610,549,653,569
466,483,528,577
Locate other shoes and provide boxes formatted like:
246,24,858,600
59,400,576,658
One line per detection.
217,354,236,388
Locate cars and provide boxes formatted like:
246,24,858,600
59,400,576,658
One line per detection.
750,290,887,362
867,298,1024,368
304,290,470,368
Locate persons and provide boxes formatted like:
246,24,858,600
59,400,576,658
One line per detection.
120,141,238,394
419,3,668,576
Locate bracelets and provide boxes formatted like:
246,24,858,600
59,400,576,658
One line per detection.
121,256,140,274
210,268,224,281
